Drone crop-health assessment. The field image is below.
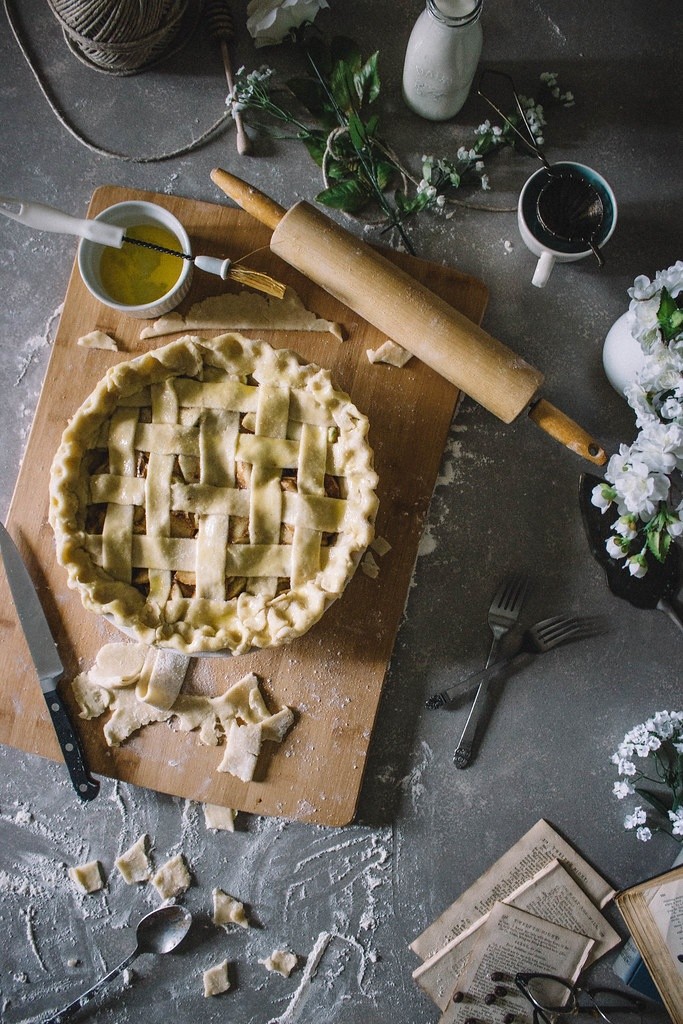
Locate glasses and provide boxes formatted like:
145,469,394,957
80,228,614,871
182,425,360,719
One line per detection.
515,972,649,1024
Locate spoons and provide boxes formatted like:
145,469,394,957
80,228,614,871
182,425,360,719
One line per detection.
41,905,192,1024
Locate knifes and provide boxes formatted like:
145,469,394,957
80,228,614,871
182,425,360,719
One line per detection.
0,521,101,801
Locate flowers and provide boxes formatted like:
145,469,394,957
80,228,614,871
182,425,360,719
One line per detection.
589,262,683,583
607,710,683,845
225,65,405,176
246,0,420,258
379,67,577,237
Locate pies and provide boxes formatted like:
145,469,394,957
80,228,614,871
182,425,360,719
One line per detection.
45,331,379,656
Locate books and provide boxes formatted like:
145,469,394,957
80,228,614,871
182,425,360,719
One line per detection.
612,847,683,1024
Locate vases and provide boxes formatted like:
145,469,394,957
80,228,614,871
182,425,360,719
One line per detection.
602,310,649,398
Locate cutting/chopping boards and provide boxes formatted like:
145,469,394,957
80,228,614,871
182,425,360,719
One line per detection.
1,183,492,825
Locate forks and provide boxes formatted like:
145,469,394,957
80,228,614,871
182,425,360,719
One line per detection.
424,611,580,711
452,571,530,769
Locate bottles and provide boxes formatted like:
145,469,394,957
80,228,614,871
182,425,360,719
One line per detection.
401,0,484,121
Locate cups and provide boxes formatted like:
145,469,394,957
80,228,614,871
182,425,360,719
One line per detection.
77,199,194,319
519,161,618,289
